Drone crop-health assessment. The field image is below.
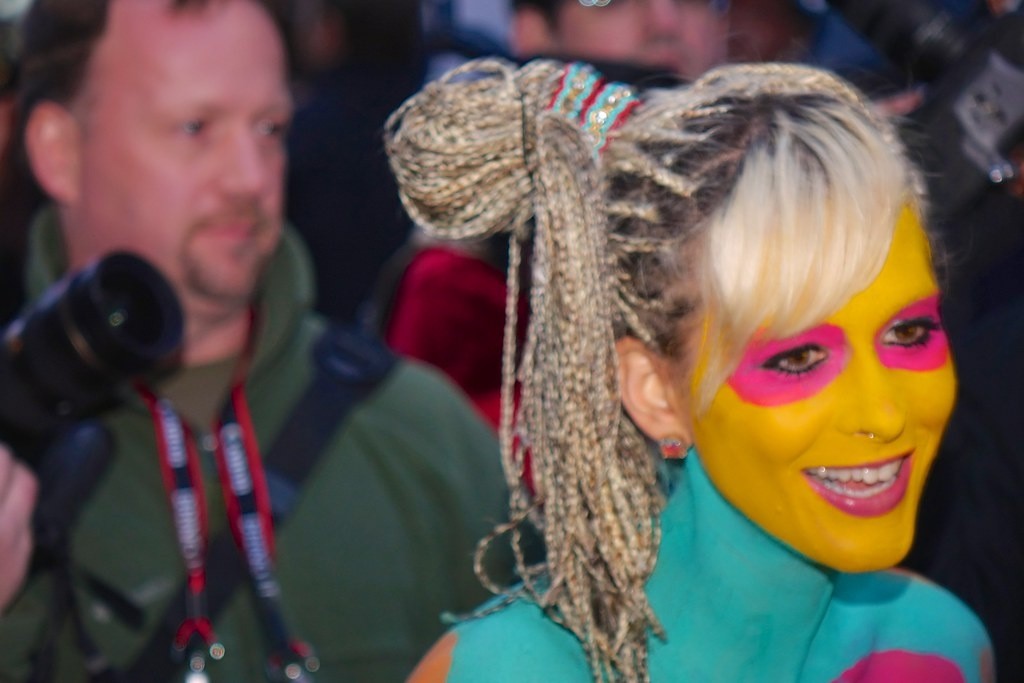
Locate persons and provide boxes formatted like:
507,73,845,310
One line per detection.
0,0,1024,498
0,0,548,683
384,57,1000,683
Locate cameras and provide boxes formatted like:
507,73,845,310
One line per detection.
0,247,184,471
